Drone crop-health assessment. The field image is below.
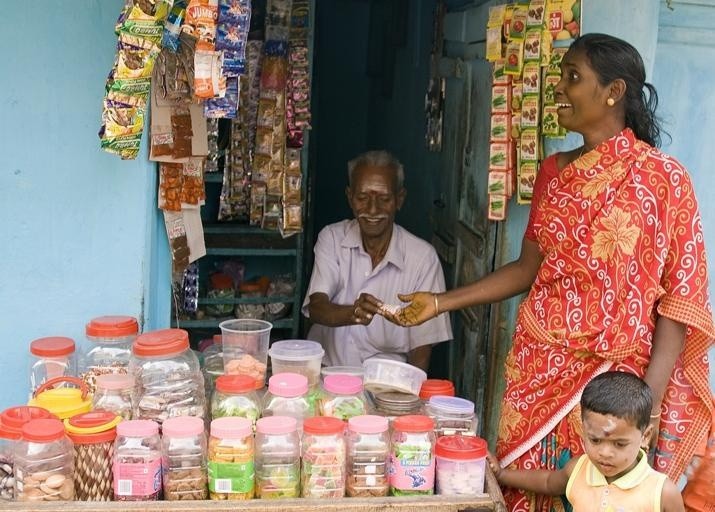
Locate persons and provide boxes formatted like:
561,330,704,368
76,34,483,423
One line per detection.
484,370,687,512
298,147,457,380
372,32,715,512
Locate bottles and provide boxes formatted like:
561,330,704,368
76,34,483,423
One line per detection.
0,406,61,503
206,417,256,502
299,417,346,501
64,409,124,502
387,415,436,499
0,315,490,436
114,420,162,501
345,414,390,498
12,419,76,502
434,436,490,495
205,273,270,321
159,416,208,502
255,416,302,501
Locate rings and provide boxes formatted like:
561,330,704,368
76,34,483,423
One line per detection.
354,306,361,314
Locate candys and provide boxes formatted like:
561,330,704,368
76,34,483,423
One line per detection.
0,355,486,501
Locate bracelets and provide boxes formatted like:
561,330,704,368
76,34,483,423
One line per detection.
648,409,663,424
433,293,440,316
